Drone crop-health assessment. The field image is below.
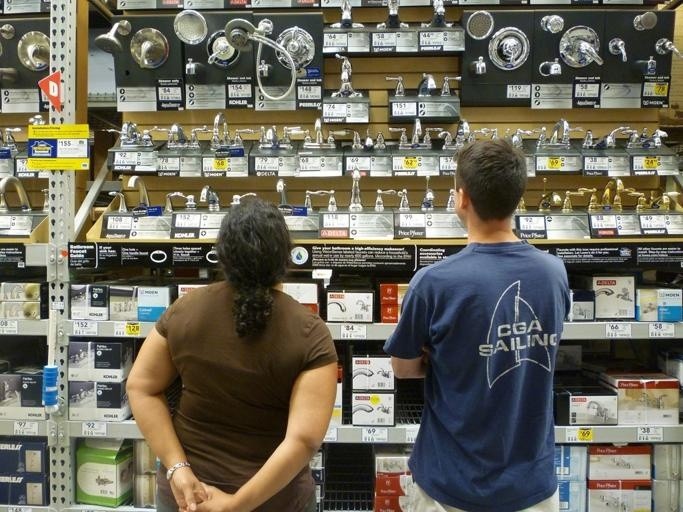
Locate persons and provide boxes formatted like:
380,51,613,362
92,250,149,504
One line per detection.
382,137,571,512
124,197,339,512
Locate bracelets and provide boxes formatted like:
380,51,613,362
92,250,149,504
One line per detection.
166,461,190,481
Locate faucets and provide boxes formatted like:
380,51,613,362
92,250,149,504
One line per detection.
0,1,670,214
327,288,657,416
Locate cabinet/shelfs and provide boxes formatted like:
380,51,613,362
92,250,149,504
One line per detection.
72,139,683,512
0,139,48,511
